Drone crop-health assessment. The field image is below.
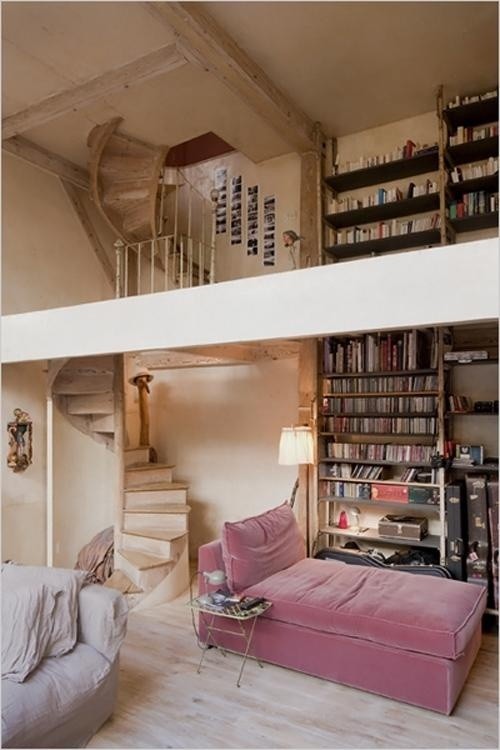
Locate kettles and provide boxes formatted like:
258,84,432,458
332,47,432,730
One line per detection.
203,569,231,607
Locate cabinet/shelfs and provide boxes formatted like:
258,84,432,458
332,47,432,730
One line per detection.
316,316,499,617
323,88,500,262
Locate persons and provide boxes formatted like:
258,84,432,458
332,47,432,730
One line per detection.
282,231,301,269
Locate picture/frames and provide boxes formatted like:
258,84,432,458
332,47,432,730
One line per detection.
214,168,276,266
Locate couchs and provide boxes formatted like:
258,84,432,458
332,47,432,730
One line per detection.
0,560,129,749
196,498,488,717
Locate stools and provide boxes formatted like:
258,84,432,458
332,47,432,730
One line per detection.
185,585,273,687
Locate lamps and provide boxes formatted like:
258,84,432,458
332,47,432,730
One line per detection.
278,424,314,467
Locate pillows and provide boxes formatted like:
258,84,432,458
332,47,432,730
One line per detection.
221,499,309,597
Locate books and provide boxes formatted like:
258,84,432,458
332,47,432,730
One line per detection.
328,481,440,504
446,88,497,149
326,442,438,485
445,155,497,220
450,393,497,416
328,178,442,248
322,328,439,394
328,395,437,435
332,139,440,175
